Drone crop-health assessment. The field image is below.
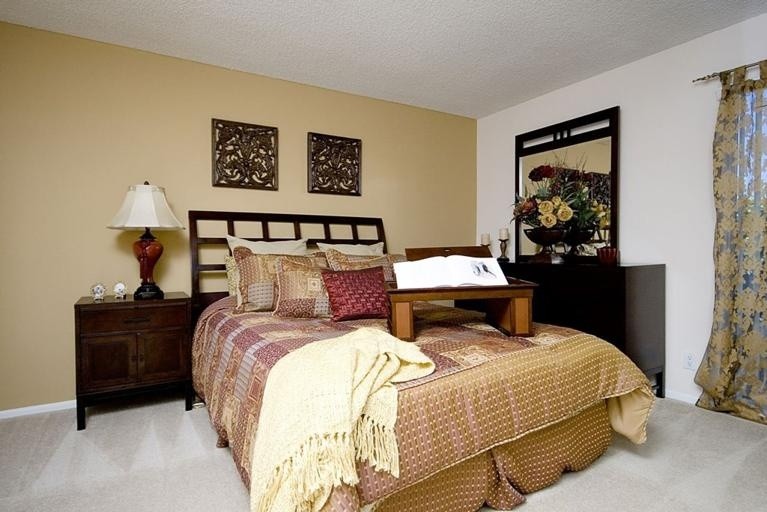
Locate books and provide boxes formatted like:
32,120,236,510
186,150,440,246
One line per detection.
392,254,510,290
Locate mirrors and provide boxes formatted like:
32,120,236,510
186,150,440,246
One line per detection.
512,105,623,265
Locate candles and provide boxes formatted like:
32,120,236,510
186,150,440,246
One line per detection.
498,227,508,240
480,231,490,244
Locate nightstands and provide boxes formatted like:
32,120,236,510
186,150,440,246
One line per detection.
71,292,192,432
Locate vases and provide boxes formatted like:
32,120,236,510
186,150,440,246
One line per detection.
520,225,563,253
561,230,594,254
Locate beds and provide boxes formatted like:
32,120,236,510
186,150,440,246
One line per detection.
184,209,656,511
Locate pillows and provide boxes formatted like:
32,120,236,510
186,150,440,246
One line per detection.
222,236,407,321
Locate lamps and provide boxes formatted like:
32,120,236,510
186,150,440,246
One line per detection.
105,179,186,299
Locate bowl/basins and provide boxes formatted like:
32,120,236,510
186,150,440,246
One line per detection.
597,247,618,264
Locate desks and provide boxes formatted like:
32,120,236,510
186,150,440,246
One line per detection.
382,273,537,342
497,262,668,399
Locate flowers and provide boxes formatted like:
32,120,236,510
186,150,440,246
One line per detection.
583,150,611,232
509,151,586,228
